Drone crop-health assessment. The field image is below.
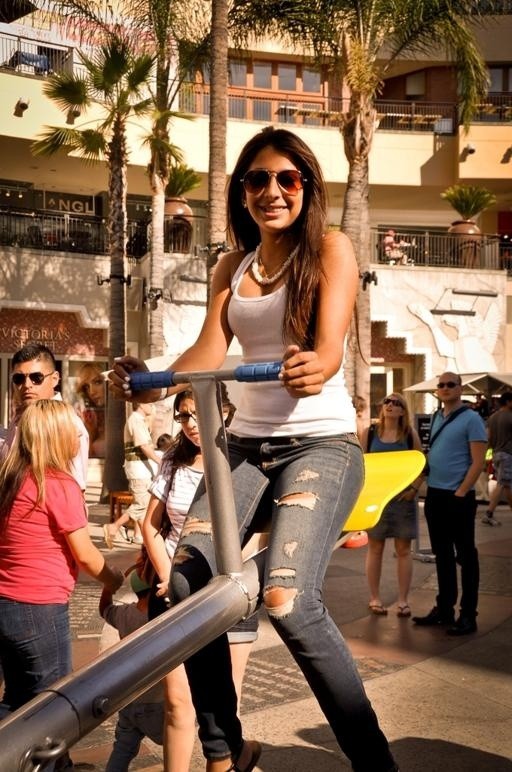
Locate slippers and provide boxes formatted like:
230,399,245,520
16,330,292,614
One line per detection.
397,605,411,616
104,523,117,549
369,605,387,615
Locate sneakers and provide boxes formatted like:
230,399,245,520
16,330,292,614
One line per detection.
481,515,501,526
119,525,129,541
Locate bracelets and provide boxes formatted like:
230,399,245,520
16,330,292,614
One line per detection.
411,487,419,492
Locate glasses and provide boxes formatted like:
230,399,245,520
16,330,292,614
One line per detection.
174,400,198,423
12,371,55,386
437,382,455,388
384,399,404,409
239,169,309,193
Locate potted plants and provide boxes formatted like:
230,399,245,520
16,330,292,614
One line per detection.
440,183,498,266
166,161,204,251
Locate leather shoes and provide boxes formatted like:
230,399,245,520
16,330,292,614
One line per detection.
446,614,477,636
412,606,455,624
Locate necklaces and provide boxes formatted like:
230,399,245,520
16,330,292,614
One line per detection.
249,243,303,288
259,257,286,282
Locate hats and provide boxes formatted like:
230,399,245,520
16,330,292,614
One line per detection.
129,571,149,594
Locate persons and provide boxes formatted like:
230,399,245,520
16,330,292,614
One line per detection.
70,360,115,456
1,399,126,771
355,394,426,621
141,379,266,771
481,393,512,528
156,435,174,457
102,392,163,552
109,125,399,771
100,555,180,771
382,228,413,269
0,346,88,510
412,372,488,634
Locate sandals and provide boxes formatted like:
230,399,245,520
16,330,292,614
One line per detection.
225,738,262,771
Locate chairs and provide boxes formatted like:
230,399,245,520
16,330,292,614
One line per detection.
27,226,57,251
376,241,400,266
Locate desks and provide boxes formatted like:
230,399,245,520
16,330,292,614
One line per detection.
275,102,511,130
